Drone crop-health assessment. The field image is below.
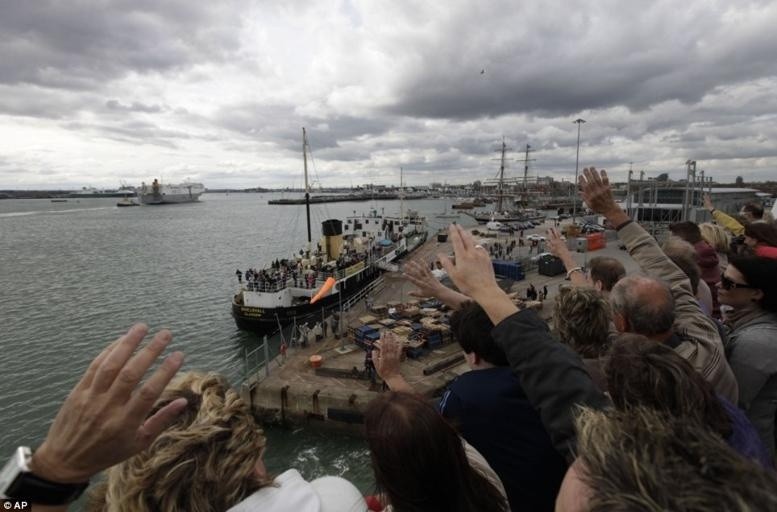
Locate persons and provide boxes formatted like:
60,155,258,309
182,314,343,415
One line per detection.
104,371,368,511
0,323,187,511
234,166,777,512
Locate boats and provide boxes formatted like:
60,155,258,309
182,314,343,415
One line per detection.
116,201,140,207
135,180,206,204
50,199,67,202
68,184,135,198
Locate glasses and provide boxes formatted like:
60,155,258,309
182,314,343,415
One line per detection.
720,272,756,290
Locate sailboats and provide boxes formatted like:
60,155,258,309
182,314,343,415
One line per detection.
229,127,428,338
451,135,585,232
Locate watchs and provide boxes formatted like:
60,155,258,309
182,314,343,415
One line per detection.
0,446,87,506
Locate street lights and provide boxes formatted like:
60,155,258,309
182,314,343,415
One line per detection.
571,117,588,226
